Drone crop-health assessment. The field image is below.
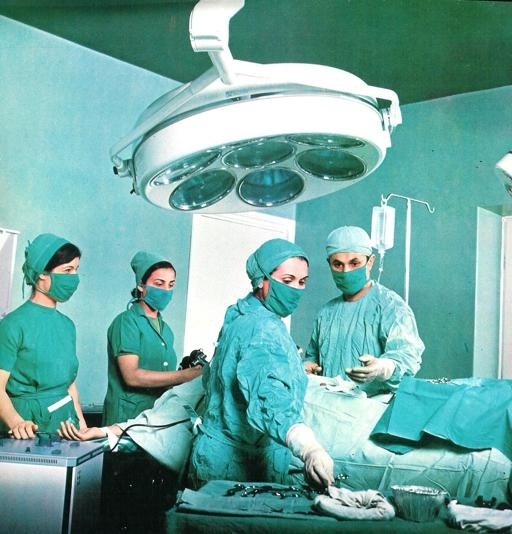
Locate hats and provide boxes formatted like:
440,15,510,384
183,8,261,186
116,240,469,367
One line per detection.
326,225,373,258
246,238,310,291
21,232,72,286
129,250,173,288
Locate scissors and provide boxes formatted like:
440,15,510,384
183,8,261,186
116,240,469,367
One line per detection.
306,366,321,375
336,472,349,482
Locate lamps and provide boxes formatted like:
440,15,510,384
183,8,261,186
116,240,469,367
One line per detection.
112,0,404,215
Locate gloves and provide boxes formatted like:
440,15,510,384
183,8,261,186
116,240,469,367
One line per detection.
287,423,336,490
344,353,397,384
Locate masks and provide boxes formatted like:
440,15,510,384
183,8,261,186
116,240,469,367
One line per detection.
141,284,174,313
329,257,372,295
38,270,80,304
263,272,306,317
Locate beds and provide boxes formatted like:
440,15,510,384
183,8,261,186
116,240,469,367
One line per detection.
305,373,511,498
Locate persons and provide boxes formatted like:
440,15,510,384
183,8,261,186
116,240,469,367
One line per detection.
100,250,205,429
300,226,426,389
184,237,335,501
1,233,88,442
